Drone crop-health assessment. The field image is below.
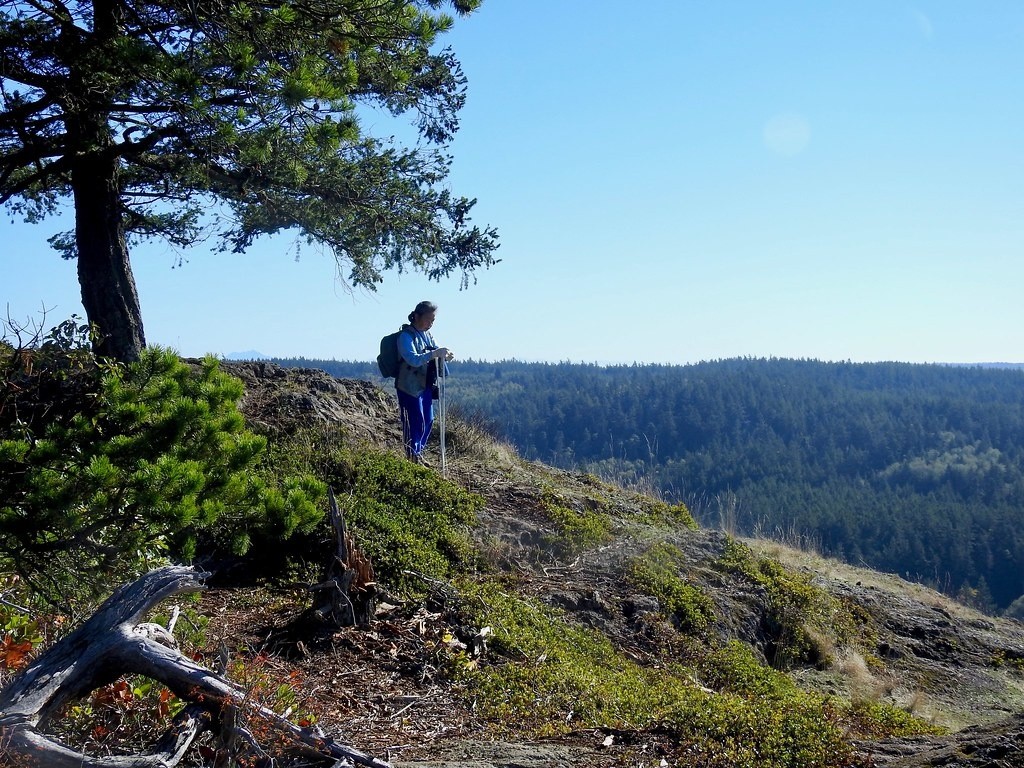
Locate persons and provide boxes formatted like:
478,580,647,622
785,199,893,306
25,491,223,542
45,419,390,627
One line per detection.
395,301,453,469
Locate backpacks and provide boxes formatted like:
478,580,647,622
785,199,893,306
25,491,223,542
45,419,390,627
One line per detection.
377,325,416,379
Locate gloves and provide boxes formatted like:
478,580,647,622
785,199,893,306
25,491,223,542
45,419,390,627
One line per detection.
445,351,454,361
434,347,449,358
